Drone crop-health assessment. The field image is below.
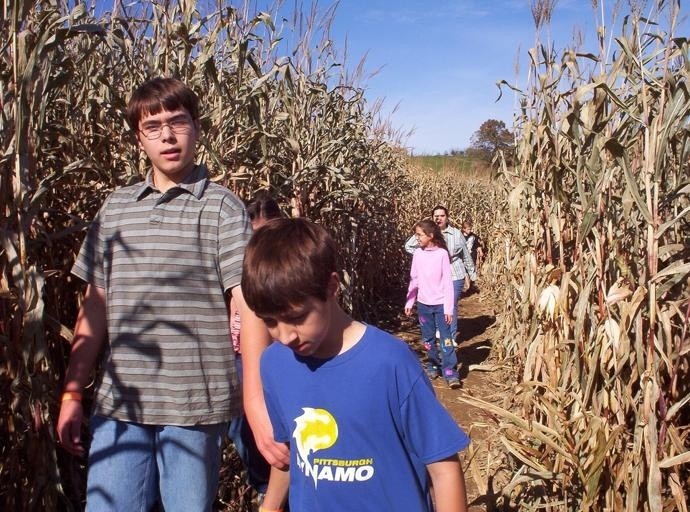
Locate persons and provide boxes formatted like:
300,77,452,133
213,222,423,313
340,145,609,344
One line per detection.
459,221,486,293
402,205,479,351
403,219,463,391
49,76,292,512
220,198,284,505
236,213,476,511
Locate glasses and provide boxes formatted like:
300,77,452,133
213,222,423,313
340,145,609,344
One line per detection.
138,117,194,137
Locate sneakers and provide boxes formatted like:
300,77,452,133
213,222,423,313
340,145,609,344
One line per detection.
445,379,461,387
427,369,439,381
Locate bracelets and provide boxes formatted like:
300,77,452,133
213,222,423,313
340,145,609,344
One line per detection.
55,390,83,402
